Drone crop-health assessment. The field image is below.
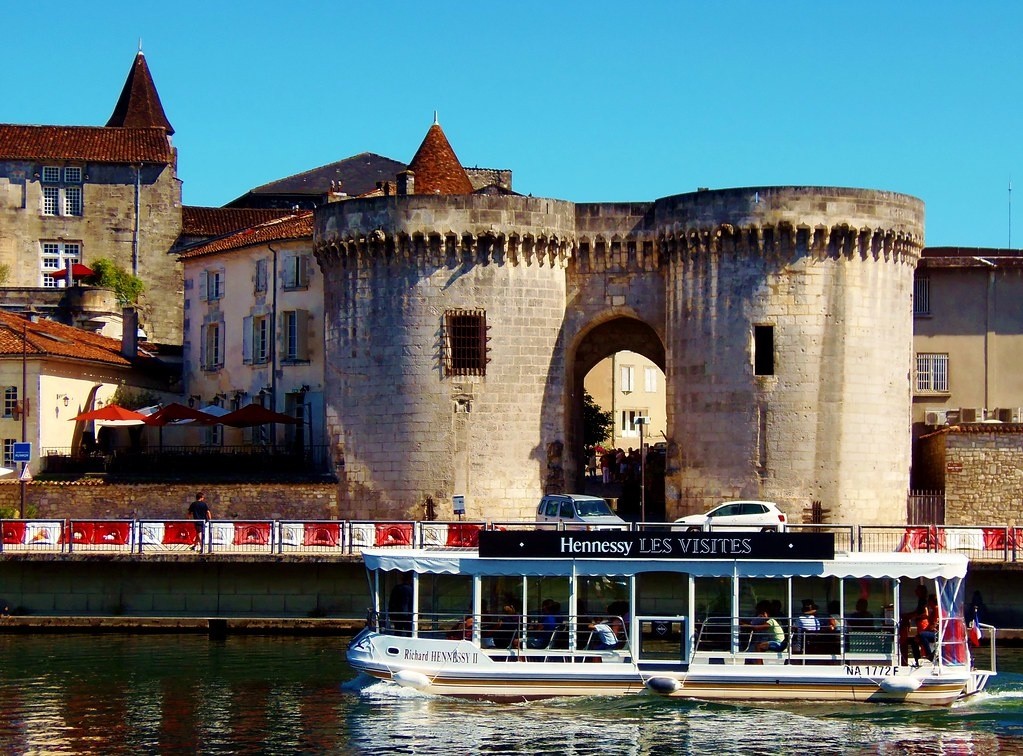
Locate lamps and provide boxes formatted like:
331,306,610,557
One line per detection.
33,166,41,180
234,391,247,404
213,393,226,406
188,395,201,408
300,384,309,398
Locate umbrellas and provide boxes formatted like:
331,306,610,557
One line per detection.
49,265,100,287
69,401,302,450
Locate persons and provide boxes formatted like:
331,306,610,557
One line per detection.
453,585,989,670
585,445,655,485
186,492,211,553
387,572,415,638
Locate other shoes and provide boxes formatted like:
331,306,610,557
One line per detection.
191,547,195,551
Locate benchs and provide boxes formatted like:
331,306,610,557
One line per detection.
707,627,795,652
543,627,629,661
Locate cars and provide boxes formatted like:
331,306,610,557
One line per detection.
670,500,790,533
653,442,667,454
535,493,630,532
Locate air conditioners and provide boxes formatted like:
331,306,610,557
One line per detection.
996,407,1020,423
925,411,946,425
259,387,272,401
959,407,984,423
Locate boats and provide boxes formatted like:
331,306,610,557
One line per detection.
343,528,999,707
